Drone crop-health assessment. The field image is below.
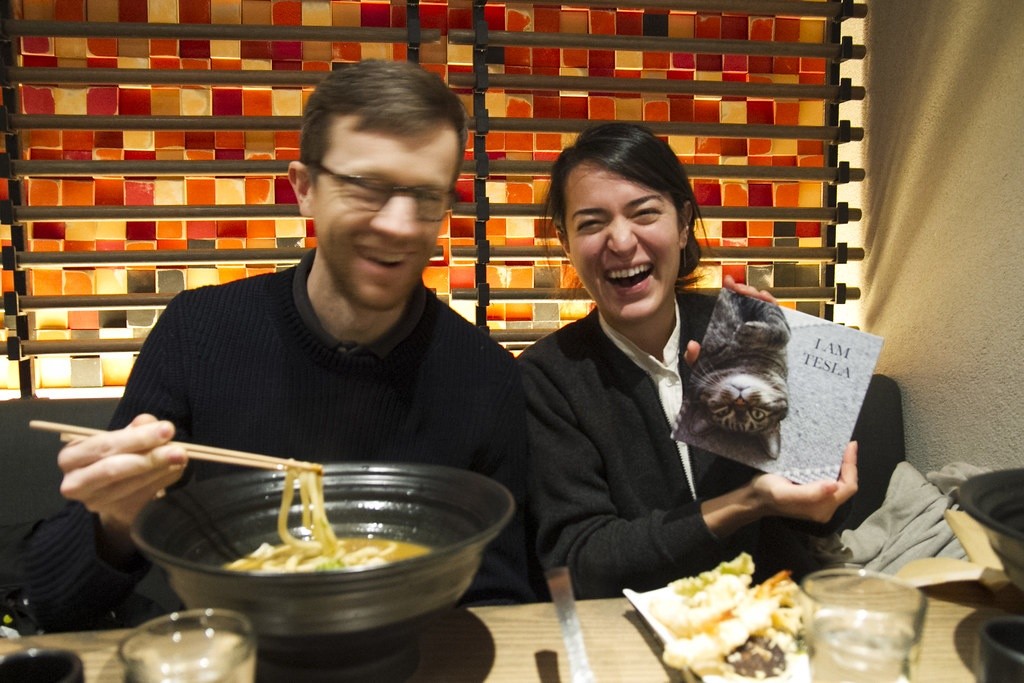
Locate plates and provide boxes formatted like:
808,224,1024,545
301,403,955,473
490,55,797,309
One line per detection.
622,581,916,683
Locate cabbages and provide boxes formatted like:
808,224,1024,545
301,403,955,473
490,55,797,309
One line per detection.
663,553,809,668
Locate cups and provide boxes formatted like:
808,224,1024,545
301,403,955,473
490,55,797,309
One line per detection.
117,608,257,683
978,616,1024,683
0,647,82,683
800,567,924,683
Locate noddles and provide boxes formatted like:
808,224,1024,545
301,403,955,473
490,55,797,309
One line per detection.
228,462,400,572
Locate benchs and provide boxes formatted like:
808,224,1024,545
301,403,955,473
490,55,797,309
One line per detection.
0,373,904,626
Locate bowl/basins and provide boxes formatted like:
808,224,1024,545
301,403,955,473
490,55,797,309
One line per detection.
130,461,514,683
959,466,1024,594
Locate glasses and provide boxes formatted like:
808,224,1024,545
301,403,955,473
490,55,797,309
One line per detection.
313,161,456,222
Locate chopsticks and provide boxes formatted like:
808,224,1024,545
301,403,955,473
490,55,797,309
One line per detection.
29,419,325,476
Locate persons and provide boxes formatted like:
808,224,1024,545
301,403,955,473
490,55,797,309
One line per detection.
515,123,857,604
20,59,535,633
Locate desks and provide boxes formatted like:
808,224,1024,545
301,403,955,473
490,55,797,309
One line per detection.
0,581,1024,683
